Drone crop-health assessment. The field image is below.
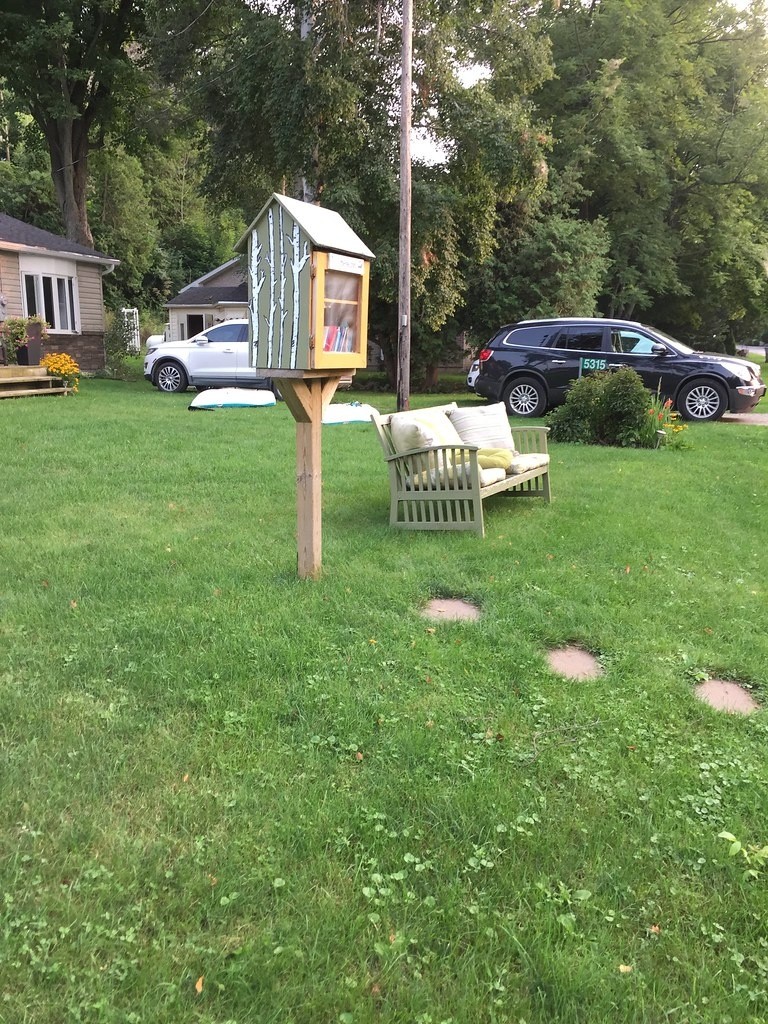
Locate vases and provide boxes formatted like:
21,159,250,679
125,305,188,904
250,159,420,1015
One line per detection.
16,322,42,365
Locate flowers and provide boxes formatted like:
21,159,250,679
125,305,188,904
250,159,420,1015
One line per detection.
39,353,80,391
3,314,52,350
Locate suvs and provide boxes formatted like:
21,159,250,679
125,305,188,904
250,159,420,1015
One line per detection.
143,318,353,394
473,318,767,423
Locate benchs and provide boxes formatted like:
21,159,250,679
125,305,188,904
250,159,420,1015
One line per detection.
370,402,551,537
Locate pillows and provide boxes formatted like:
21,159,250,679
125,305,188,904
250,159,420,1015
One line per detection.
446,401,516,451
450,446,513,469
388,409,465,471
